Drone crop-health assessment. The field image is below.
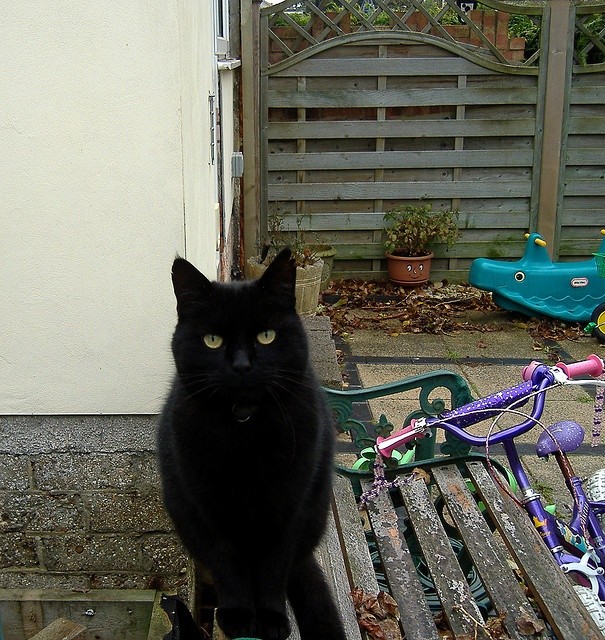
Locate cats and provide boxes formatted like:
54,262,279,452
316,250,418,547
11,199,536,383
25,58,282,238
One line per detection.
156,244,348,640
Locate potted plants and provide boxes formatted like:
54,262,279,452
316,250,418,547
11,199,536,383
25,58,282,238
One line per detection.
247,213,323,317
290,216,336,292
382,206,463,285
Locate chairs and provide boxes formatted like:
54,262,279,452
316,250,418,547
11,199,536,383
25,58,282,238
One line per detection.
162,368,605,639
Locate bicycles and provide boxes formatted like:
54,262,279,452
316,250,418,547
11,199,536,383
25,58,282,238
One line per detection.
375,354,605,635
